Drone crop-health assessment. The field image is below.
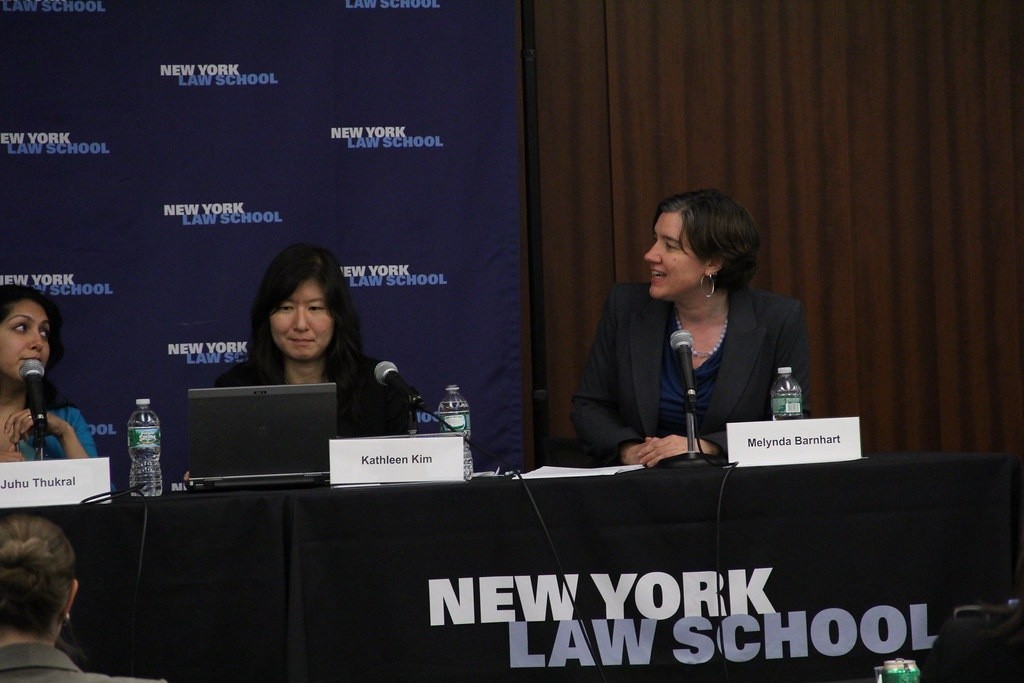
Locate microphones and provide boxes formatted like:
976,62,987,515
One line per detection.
18,358,50,437
670,329,698,413
373,360,431,416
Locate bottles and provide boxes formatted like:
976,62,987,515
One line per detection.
437,384,473,482
770,366,803,422
127,397,163,497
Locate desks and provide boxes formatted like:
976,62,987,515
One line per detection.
1,452,1024,683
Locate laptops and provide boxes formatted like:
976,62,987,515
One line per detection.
185,383,336,492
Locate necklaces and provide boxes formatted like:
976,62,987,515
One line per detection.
671,302,730,357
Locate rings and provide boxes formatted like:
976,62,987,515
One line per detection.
27,414,32,419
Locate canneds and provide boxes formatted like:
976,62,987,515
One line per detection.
881,658,920,683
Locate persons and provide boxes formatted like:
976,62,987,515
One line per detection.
916,546,1024,683
564,188,811,466
0,283,116,498
0,514,169,682
180,243,413,485
